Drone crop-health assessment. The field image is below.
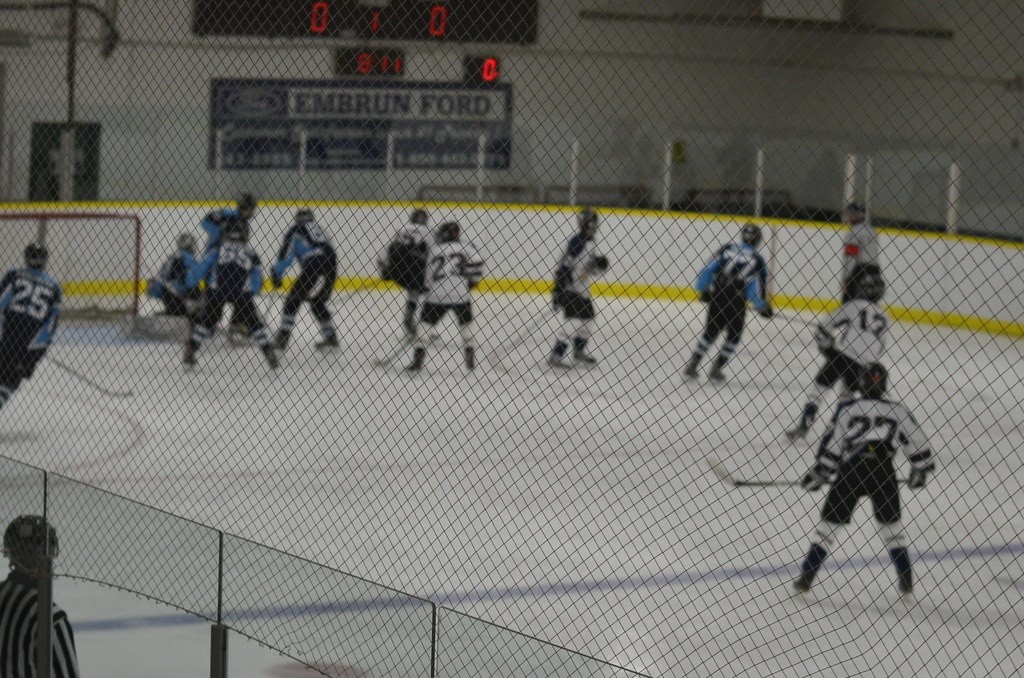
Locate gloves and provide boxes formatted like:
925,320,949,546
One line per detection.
272,272,281,287
701,290,711,300
909,471,926,487
761,308,773,318
596,256,608,270
802,472,827,490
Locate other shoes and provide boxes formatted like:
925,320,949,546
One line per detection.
710,369,724,380
183,326,478,373
788,426,807,438
901,580,913,592
549,357,570,368
573,352,595,363
686,366,698,377
794,579,810,592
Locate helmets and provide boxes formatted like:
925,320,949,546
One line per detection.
239,193,255,218
3,515,59,573
858,273,885,297
226,221,248,243
579,210,597,233
297,210,313,222
847,202,866,222
861,361,888,397
178,233,194,249
440,223,459,240
25,243,47,270
742,225,762,247
412,210,427,224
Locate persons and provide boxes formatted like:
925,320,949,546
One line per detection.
794,363,935,592
381,209,440,338
270,210,338,350
0,515,83,677
547,208,608,365
0,242,61,409
684,225,774,377
842,203,879,300
406,222,482,370
787,279,888,439
146,193,278,369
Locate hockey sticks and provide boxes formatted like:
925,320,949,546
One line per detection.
700,293,822,328
151,273,314,348
42,351,135,399
329,278,383,305
372,332,416,367
489,265,595,358
705,455,911,489
